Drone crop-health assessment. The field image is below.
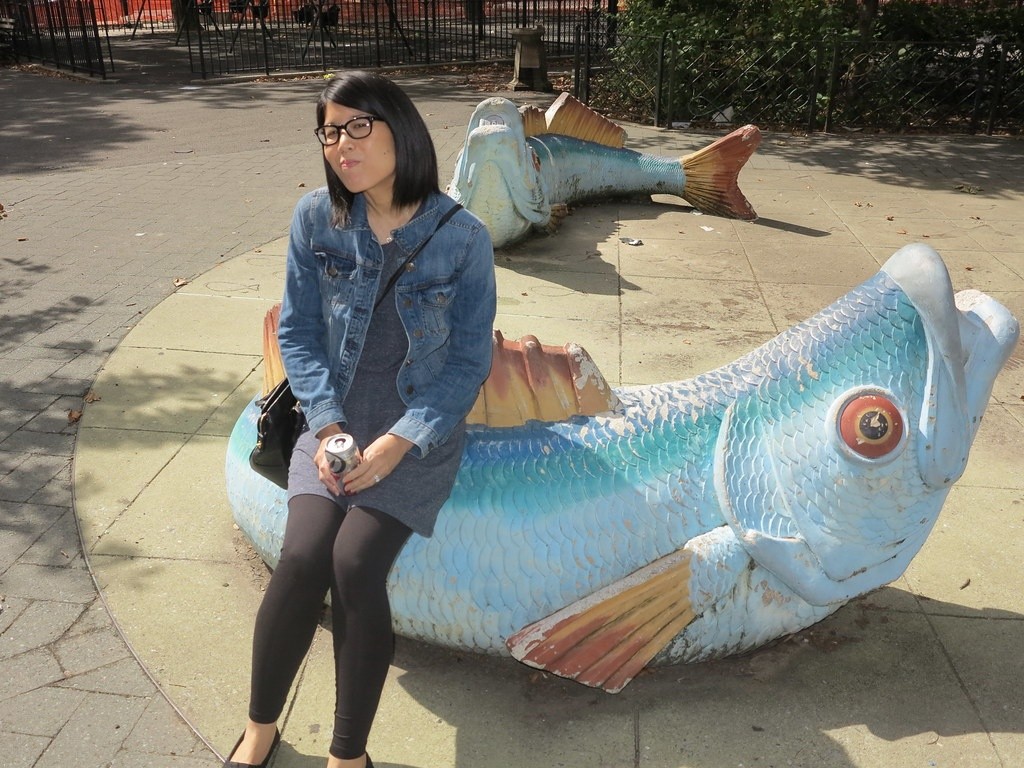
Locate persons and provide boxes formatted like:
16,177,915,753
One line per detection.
219,70,497,768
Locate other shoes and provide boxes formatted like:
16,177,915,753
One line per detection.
363,751,374,768
221,724,280,768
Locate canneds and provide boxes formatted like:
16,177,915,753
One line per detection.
324,433,363,496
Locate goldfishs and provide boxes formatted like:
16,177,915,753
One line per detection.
223,245,1019,695
447,86,761,249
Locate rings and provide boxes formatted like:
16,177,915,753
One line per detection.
373,475,381,484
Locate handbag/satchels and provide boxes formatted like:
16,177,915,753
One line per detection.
248,380,309,490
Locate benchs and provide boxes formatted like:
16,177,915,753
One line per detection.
0,17,20,65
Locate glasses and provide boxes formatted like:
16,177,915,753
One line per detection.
313,116,385,146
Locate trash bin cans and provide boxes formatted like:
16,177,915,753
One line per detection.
171,0,204,31
506,29,554,91
